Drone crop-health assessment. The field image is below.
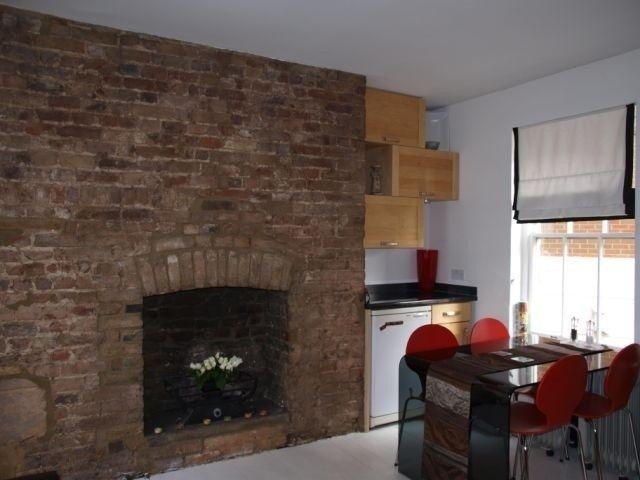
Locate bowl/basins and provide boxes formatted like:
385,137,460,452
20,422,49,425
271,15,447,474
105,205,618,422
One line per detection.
425,141,441,150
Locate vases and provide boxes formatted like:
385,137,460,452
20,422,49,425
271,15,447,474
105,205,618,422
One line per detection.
204,398,229,418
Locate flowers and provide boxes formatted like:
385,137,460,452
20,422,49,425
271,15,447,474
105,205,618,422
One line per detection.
189,348,244,396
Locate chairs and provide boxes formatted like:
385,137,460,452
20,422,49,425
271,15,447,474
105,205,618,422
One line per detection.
506,342,640,479
406,317,510,353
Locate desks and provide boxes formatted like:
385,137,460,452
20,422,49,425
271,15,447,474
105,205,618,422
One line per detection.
397,341,613,480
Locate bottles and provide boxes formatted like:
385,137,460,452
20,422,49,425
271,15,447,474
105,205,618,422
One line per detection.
515,301,529,346
371,161,384,196
417,250,438,291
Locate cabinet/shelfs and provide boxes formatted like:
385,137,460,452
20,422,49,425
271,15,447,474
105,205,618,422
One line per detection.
365,86,461,251
430,301,471,352
